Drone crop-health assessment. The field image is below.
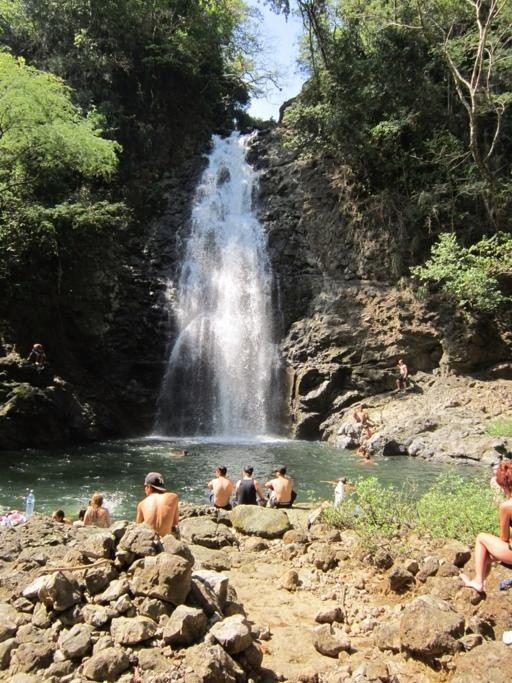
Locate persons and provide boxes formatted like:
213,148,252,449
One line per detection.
261,466,297,507
51,506,74,527
393,359,410,393
136,470,181,544
353,403,374,465
460,460,512,597
501,624,512,648
83,493,112,528
29,341,48,366
333,475,348,509
206,464,235,512
72,509,85,528
180,447,191,458
233,465,264,504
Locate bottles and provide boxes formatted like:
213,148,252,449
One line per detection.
498,578,512,591
25,489,34,516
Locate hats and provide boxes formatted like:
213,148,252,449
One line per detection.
244,465,253,475
144,472,167,492
274,465,287,475
